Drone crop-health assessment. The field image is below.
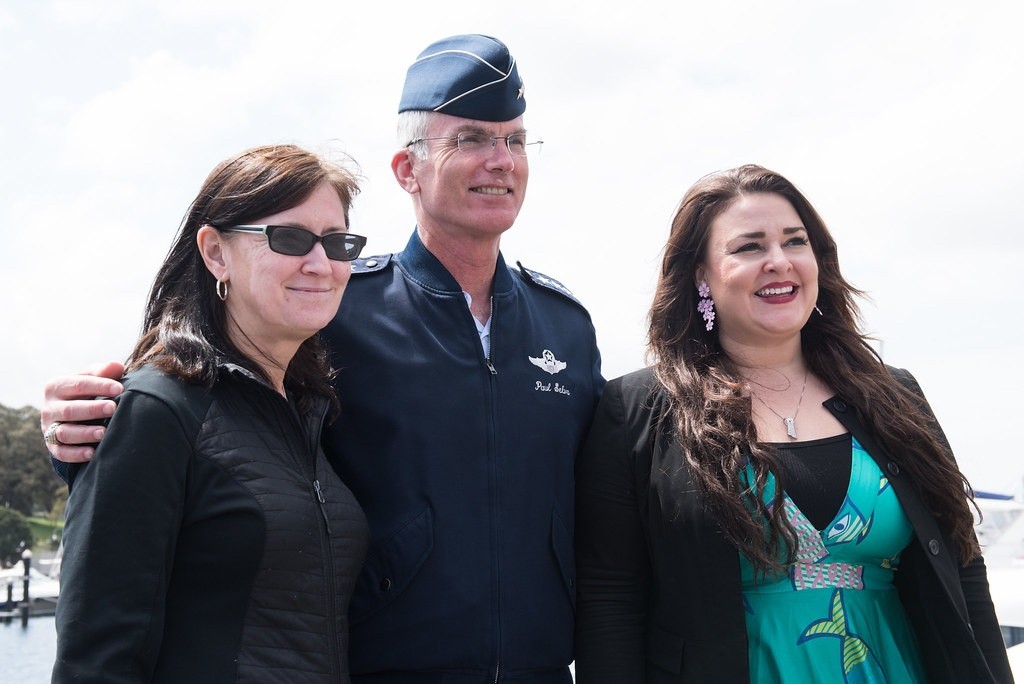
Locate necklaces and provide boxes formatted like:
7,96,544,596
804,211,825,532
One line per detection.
736,357,811,442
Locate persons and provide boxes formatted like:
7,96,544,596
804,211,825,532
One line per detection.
573,162,1018,684
40,31,608,684
50,145,376,683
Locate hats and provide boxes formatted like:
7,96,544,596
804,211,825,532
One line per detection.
399,35,526,121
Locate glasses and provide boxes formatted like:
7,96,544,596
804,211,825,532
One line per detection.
407,131,543,157
218,223,366,261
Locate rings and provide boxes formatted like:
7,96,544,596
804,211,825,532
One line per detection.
43,419,65,445
